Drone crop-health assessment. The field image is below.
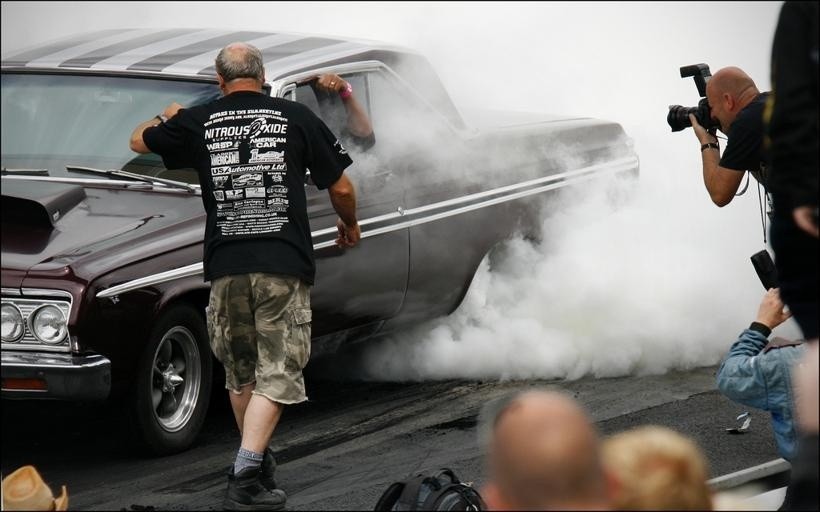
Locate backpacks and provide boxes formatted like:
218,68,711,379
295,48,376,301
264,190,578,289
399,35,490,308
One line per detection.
380,468,481,512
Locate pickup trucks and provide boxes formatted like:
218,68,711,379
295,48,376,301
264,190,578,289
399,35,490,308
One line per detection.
1,29,643,456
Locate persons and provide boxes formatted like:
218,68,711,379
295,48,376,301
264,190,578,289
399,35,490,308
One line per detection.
713,287,820,468
128,41,362,511
314,72,377,156
476,382,621,511
687,65,776,249
775,342,820,511
600,423,717,511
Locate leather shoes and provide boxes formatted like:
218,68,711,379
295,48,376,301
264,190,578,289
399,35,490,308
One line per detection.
222,463,288,510
260,447,277,489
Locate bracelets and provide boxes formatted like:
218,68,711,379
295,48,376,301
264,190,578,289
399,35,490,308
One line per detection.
154,112,171,127
700,142,721,152
340,79,353,98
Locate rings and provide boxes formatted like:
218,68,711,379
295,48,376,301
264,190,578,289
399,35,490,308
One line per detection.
330,81,336,86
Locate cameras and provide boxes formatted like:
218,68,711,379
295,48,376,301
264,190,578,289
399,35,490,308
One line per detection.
375,469,489,512
666,61,719,140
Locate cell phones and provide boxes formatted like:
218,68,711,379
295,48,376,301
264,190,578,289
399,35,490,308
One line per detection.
750,249,784,293
